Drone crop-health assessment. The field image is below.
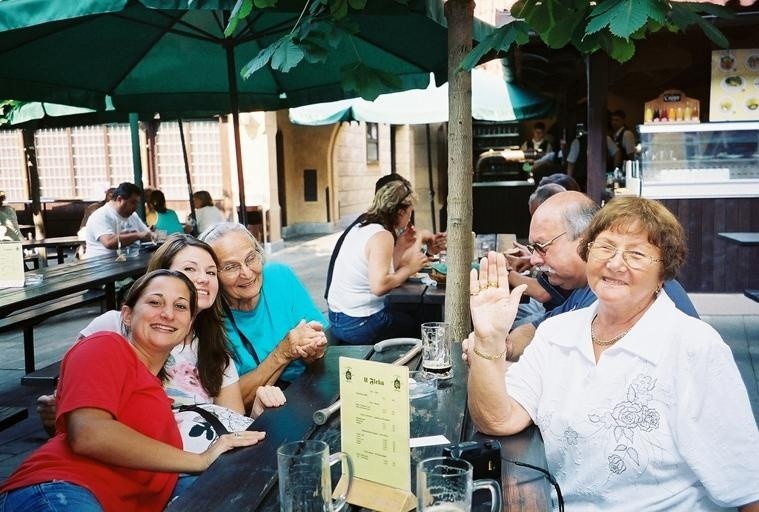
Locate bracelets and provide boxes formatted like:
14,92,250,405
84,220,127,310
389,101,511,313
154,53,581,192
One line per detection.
472,348,510,362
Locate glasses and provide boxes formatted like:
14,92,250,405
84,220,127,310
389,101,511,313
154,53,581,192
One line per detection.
587,242,665,270
396,185,411,208
219,250,262,275
526,231,567,257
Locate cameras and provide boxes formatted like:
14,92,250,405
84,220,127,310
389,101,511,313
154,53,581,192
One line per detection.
442,440,502,493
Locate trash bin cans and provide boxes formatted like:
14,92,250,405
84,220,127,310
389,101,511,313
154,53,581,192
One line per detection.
238,206,262,242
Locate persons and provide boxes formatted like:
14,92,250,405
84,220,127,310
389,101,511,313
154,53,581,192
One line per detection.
74,232,289,498
1,268,266,512
193,221,336,417
75,179,230,294
505,108,639,321
322,171,449,304
467,194,758,512
327,181,429,345
460,189,702,371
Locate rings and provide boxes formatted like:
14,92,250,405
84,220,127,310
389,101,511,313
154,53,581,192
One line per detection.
469,293,478,296
234,433,244,441
488,282,497,287
479,284,488,289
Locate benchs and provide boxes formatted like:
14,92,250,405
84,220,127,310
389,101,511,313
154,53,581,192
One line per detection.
1,222,151,430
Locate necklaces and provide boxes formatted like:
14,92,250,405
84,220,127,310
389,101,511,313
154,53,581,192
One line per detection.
591,312,638,347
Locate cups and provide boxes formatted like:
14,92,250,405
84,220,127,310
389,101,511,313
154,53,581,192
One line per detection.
475,125,519,135
420,320,454,379
410,232,491,285
413,455,502,512
151,229,168,245
275,439,353,511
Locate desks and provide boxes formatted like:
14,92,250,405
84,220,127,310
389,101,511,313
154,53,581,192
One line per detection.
385,228,518,317
162,339,557,512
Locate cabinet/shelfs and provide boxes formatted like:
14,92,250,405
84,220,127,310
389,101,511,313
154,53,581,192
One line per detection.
612,120,759,291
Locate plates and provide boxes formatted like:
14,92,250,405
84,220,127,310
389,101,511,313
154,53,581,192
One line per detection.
689,152,759,159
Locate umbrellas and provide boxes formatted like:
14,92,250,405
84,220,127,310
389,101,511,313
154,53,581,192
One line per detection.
286,27,624,124
0,0,523,225
0,88,227,220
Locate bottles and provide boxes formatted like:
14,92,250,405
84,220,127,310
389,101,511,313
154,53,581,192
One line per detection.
607,167,626,193
646,102,697,123
